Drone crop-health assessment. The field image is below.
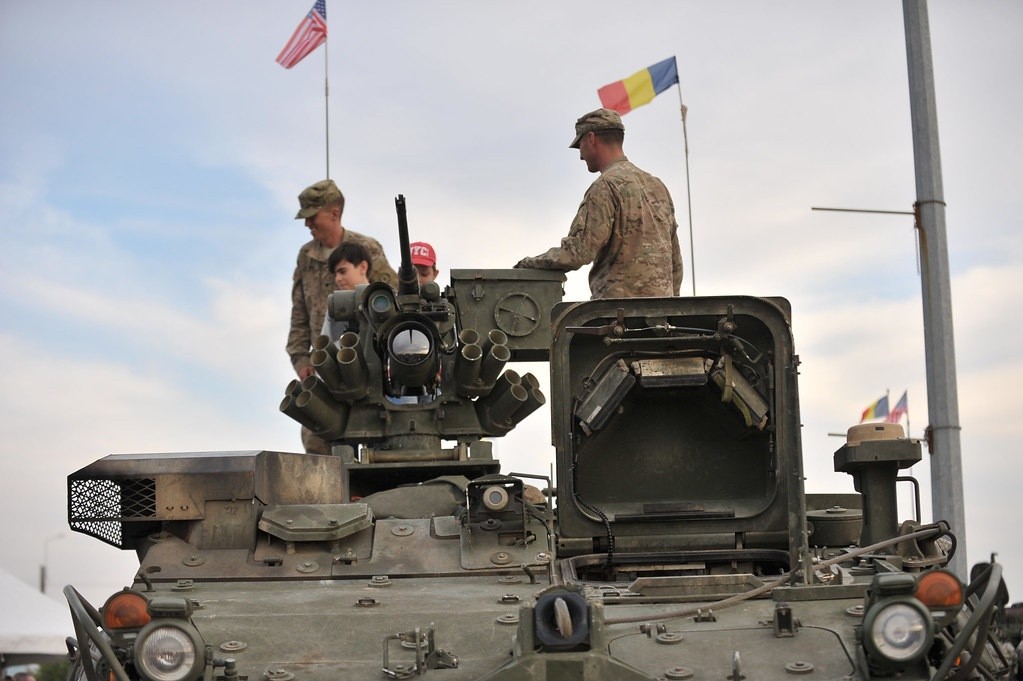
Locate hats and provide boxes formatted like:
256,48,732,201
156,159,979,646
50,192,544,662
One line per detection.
409,242,436,267
295,180,341,219
569,108,624,148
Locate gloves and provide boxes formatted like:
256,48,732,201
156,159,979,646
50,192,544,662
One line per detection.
513,257,534,268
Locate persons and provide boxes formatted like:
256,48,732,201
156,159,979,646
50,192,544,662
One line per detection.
409,242,439,284
285,178,399,455
512,107,684,299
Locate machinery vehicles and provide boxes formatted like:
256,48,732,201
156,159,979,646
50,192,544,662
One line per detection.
1,194,1023,679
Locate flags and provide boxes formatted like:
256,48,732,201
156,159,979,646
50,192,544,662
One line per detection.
274,0,328,70
597,56,680,117
860,391,908,423
